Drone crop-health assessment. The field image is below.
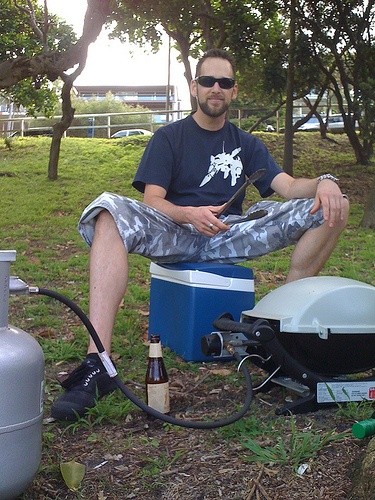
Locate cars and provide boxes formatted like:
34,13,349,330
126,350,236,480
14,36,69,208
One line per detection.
110,128,154,139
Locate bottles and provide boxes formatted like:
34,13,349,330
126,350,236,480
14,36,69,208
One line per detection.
145,334,171,415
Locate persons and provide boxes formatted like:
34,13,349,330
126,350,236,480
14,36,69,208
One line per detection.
51,48,349,422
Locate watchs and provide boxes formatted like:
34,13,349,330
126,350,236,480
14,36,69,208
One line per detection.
316,174,339,184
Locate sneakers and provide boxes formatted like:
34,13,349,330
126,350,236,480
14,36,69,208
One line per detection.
49,352,121,421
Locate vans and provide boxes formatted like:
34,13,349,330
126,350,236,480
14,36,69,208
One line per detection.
8,126,66,138
264,113,359,133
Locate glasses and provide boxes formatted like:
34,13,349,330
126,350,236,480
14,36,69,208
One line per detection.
193,74,237,90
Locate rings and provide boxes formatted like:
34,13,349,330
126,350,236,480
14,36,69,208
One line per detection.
209,223,213,229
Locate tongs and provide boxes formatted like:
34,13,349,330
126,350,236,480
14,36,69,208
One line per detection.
215,168,268,225
